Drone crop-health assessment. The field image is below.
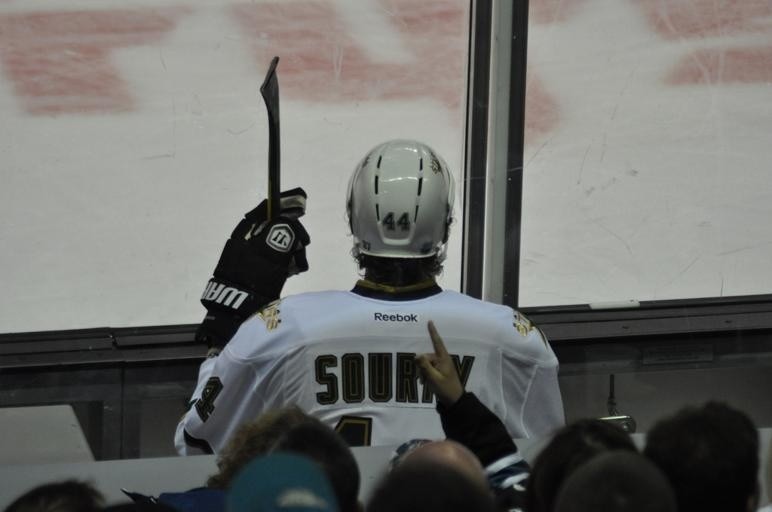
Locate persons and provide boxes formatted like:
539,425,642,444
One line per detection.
366,320,762,512
2,407,360,512
172,139,567,457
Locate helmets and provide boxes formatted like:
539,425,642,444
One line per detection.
347,140,456,259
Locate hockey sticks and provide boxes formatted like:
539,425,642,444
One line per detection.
259,58,281,221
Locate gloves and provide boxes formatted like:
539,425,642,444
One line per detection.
200,186,310,313
